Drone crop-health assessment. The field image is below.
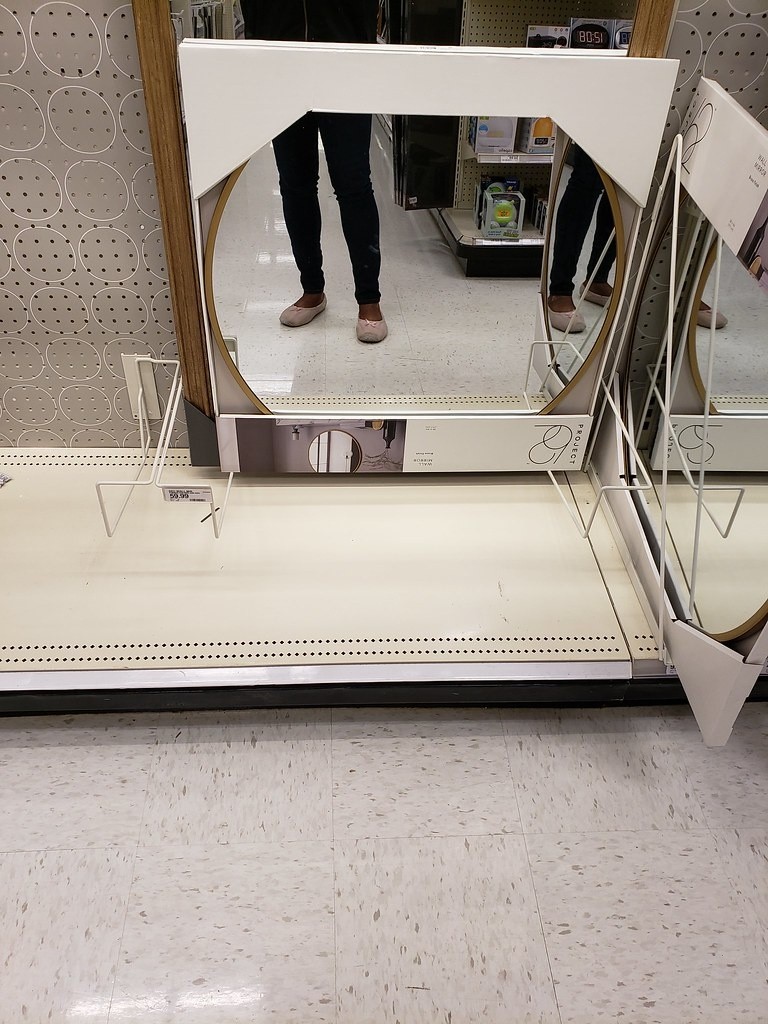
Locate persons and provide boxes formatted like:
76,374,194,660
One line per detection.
696,298,727,329
238,0,390,344
546,141,615,334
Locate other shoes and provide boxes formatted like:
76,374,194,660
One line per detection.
280,294,327,326
580,282,611,306
356,304,387,341
548,296,586,332
697,300,728,328
